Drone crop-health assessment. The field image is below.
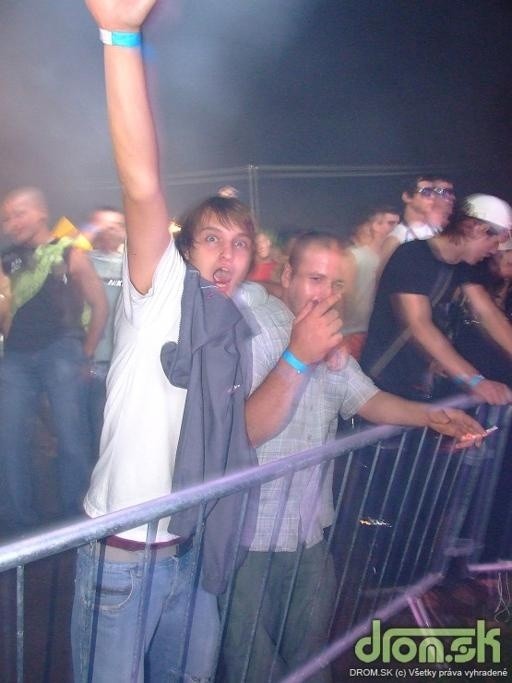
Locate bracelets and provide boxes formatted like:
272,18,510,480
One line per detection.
96,27,144,47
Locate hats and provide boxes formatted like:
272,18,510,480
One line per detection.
461,194,511,228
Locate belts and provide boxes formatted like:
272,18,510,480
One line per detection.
80,533,195,564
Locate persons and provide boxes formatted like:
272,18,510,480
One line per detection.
0,169,512,683
70,0,258,682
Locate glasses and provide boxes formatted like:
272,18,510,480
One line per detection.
412,186,455,199
